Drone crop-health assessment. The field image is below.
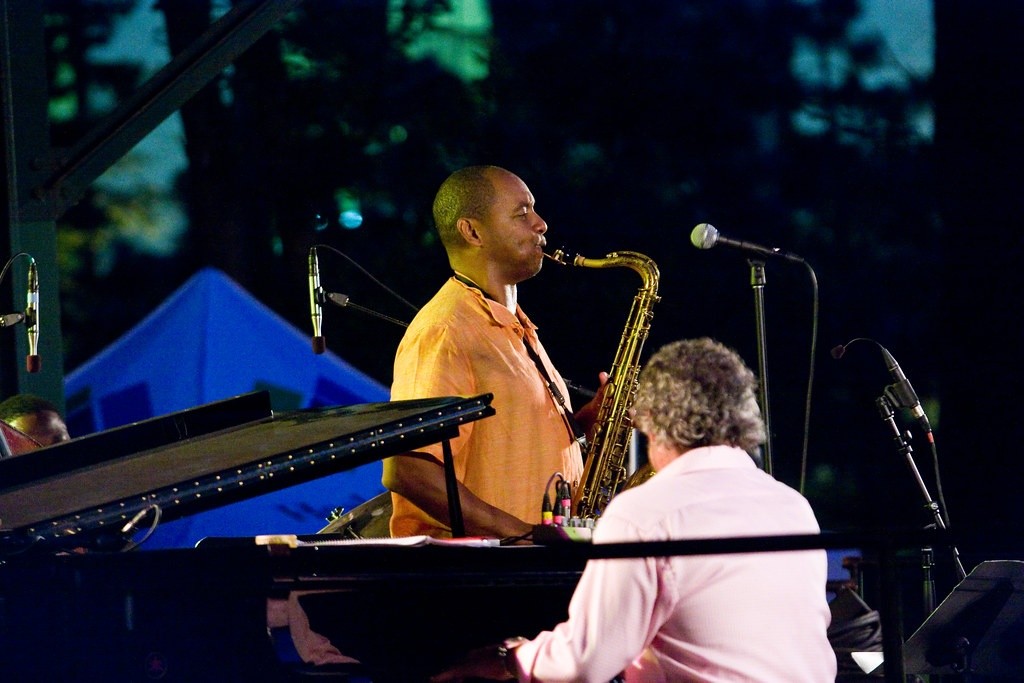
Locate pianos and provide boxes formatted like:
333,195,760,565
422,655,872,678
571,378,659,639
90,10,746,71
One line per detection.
0,389,600,683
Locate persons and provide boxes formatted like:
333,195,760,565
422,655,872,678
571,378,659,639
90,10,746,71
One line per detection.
382,165,637,546
0,395,137,555
504,338,838,683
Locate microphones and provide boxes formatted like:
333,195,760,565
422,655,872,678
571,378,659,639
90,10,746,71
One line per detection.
690,224,805,264
831,337,932,433
309,247,326,354
121,509,150,534
27,258,40,374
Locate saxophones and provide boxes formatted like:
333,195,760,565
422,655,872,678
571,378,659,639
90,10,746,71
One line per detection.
537,242,663,527
